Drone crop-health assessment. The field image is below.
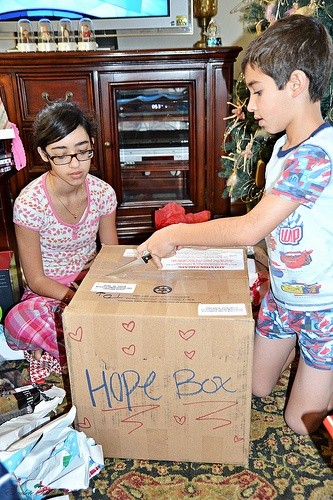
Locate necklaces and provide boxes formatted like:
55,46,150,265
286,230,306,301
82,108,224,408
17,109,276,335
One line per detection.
48,174,84,220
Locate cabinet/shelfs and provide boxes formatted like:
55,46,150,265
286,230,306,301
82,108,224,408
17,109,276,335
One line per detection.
0,46,255,271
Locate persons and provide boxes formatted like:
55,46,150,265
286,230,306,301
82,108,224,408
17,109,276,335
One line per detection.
6,102,117,364
135,13,333,436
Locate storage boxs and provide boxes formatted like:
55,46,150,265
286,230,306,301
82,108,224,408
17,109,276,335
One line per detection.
62,244,255,467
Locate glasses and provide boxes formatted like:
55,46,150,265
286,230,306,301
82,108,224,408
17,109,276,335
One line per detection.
43,143,94,165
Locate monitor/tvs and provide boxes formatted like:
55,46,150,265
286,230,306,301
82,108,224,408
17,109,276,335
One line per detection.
0,0,194,50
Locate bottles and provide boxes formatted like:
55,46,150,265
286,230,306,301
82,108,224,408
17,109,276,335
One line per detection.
206,19,222,48
